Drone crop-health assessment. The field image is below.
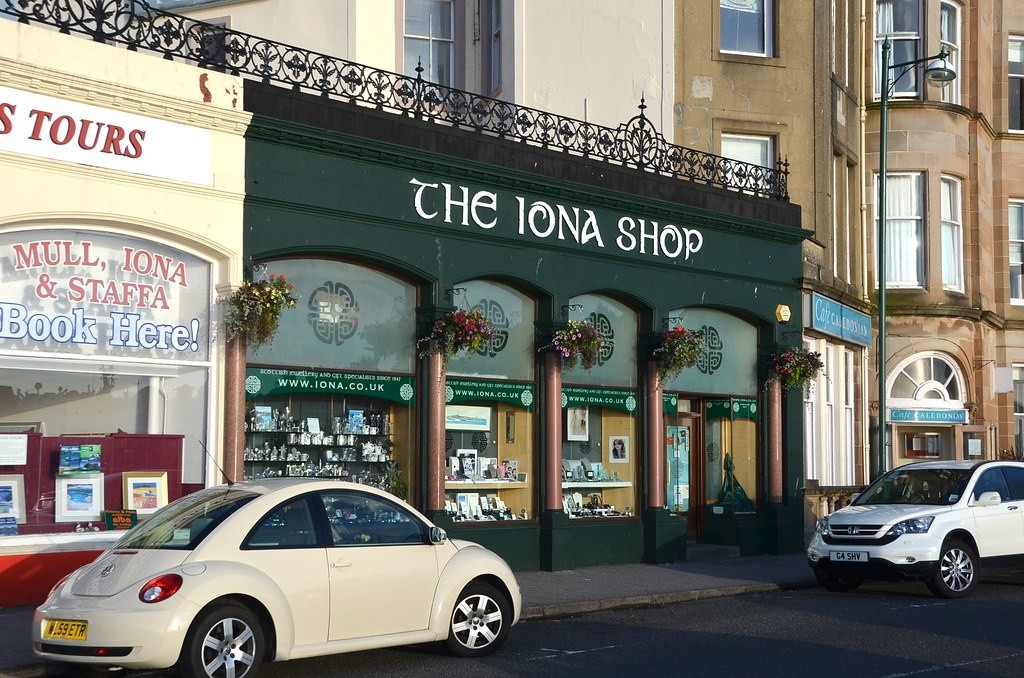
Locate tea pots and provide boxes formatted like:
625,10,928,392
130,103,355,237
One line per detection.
361,441,377,454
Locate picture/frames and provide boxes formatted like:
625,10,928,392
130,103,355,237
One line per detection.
52,471,106,523
121,468,171,519
0,471,28,524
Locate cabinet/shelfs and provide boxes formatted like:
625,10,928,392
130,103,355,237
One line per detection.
245,430,400,483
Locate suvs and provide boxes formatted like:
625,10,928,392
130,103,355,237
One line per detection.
808,460,1024,599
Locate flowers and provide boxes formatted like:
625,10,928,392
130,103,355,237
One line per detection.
539,318,607,372
417,306,495,365
210,268,305,353
761,340,833,400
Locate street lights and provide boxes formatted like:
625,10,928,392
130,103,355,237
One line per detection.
877,32,961,474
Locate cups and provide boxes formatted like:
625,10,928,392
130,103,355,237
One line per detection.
343,422,379,435
287,432,358,446
301,454,310,461
361,446,387,462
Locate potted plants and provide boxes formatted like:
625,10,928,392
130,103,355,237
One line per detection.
647,326,712,392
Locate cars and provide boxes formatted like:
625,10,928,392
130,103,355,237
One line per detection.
31,475,523,678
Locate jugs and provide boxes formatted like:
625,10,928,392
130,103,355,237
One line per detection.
330,416,345,434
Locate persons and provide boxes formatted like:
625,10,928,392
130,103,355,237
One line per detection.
850,485,866,503
572,405,587,435
613,439,626,459
938,472,989,504
